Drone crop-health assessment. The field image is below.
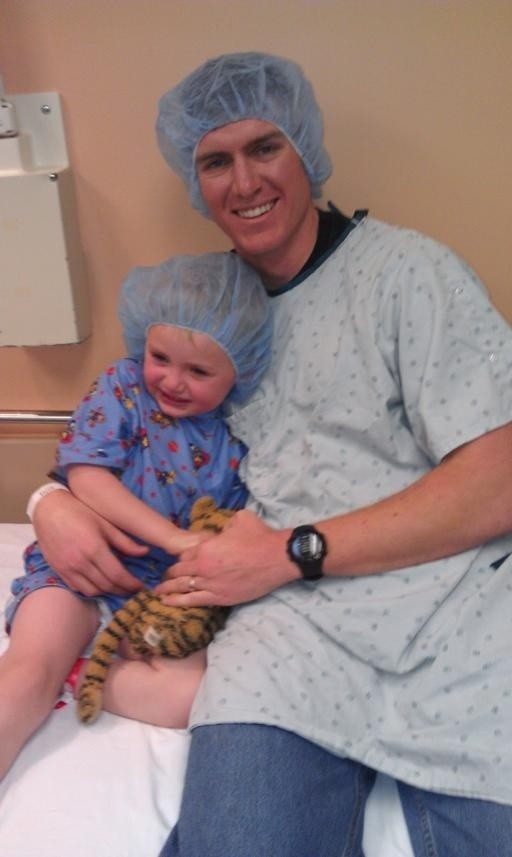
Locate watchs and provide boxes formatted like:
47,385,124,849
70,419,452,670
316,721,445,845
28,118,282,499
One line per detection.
286,525,328,581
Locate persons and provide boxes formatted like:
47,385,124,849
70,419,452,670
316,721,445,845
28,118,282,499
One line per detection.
31,51,512,857
1,251,273,787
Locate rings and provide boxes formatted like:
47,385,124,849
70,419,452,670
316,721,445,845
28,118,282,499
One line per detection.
187,575,196,591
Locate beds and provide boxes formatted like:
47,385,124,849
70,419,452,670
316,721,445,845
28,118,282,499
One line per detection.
1,411,412,857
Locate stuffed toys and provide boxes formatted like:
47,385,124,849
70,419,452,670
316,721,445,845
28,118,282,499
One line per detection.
74,494,238,727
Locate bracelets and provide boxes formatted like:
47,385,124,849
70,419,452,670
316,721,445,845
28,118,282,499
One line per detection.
25,481,70,522
